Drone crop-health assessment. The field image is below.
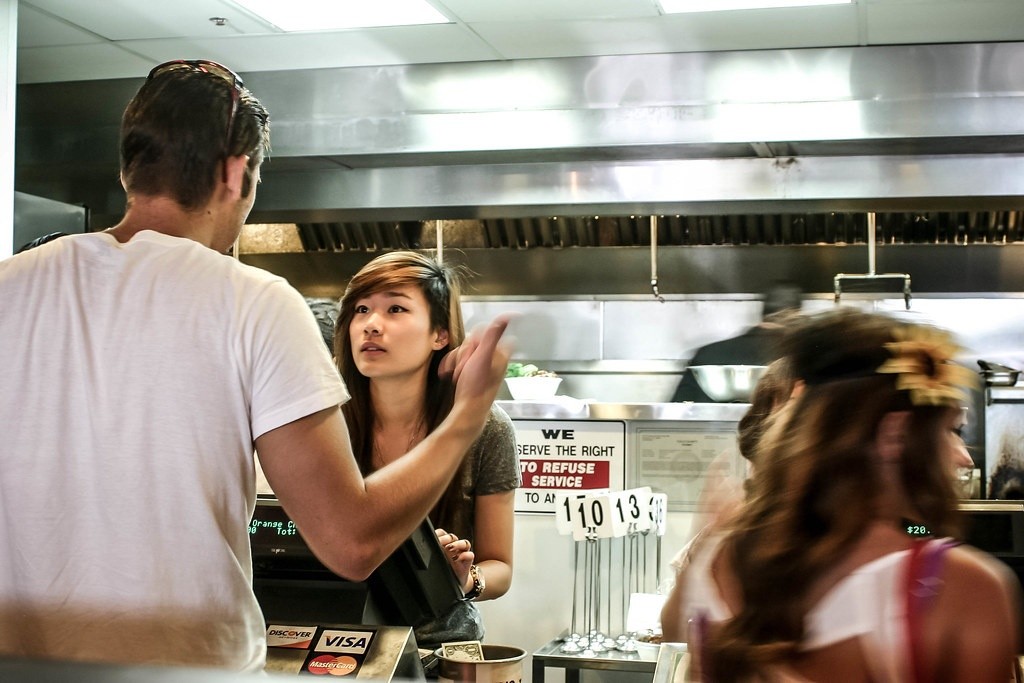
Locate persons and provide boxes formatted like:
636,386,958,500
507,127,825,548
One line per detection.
1,58,512,669
661,313,1019,682
670,286,802,402
333,252,521,650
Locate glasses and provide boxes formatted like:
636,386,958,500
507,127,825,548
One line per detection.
144,60,244,182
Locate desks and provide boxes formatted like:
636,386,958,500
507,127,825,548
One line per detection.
531,627,657,682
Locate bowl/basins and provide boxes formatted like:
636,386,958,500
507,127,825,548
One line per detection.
633,638,660,660
978,370,1022,386
505,376,562,401
687,365,768,401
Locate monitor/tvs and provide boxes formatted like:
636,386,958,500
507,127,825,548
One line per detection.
248,494,485,652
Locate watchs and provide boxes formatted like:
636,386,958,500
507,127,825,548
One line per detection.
464,565,487,599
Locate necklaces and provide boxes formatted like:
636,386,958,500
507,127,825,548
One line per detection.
371,415,424,466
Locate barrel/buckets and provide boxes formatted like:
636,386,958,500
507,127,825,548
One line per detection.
436,646,526,683
